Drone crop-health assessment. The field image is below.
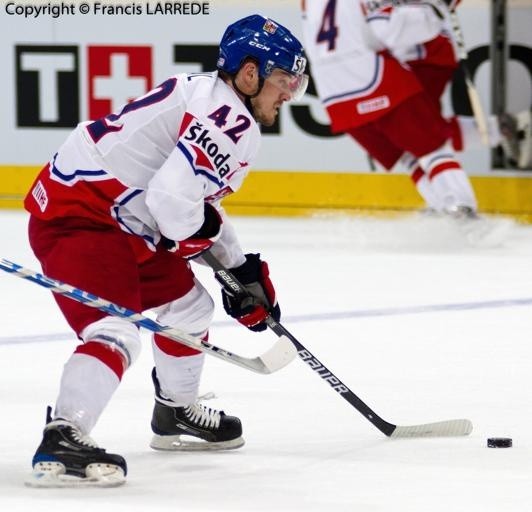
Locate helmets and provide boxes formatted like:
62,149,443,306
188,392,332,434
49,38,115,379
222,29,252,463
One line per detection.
216,14,309,104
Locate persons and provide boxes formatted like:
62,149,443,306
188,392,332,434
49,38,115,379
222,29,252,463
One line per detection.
407,0,532,169
24,13,313,479
303,2,480,215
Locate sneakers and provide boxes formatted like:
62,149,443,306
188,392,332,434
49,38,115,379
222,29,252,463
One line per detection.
150,367,242,443
33,405,127,476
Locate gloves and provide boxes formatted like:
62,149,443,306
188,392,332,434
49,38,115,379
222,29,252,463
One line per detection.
166,203,224,258
224,253,281,333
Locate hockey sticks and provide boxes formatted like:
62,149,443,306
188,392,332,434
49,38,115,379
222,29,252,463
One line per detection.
201,251,474,438
0,259,298,375
444,0,531,151
